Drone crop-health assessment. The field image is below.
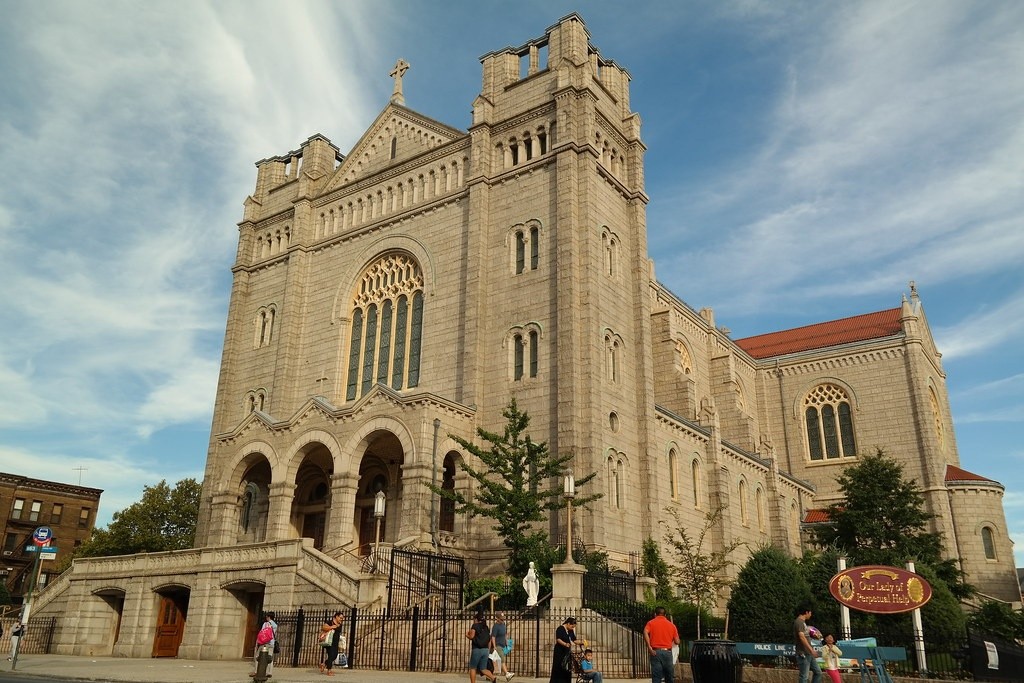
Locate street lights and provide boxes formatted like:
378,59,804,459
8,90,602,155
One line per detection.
369,490,387,573
561,467,576,564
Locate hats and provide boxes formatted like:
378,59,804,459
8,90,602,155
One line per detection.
495,612,505,619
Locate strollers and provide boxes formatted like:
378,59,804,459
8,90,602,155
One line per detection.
568,643,591,683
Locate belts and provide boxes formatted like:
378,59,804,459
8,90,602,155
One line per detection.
653,648,670,651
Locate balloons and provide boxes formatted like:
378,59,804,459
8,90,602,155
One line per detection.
807,626,822,640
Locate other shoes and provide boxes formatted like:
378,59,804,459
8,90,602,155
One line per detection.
321,663,325,674
505,672,515,682
327,671,334,676
266,674,272,678
7,657,12,662
248,672,257,677
493,676,498,683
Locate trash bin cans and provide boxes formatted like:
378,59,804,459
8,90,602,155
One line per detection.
690,639,743,683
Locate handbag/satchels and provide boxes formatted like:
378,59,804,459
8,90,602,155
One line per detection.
319,621,336,647
672,641,680,666
273,640,280,654
334,652,347,667
257,622,274,646
563,652,573,671
489,649,501,674
338,635,346,650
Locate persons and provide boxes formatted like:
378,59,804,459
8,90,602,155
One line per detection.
466,613,497,683
793,603,822,683
320,613,344,676
249,612,278,678
490,611,515,682
643,606,680,683
549,617,581,683
7,616,27,662
822,633,843,683
581,649,603,683
